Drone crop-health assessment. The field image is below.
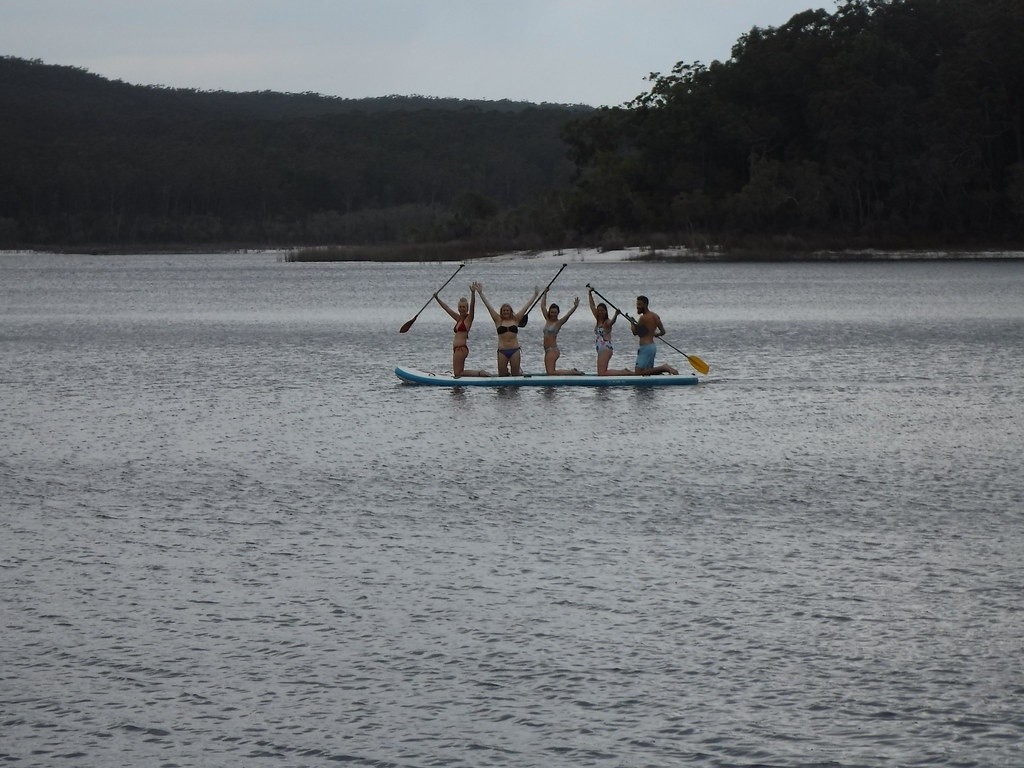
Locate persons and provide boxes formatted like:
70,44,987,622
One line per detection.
589,287,637,376
630,295,679,375
474,282,539,377
433,281,493,377
540,287,585,376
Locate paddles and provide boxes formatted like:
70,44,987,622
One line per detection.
399,263,465,334
517,262,568,328
585,282,636,326
625,312,710,375
